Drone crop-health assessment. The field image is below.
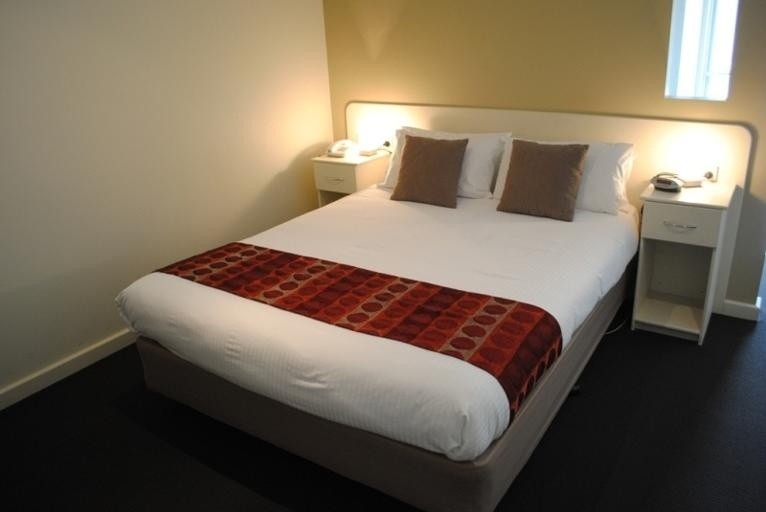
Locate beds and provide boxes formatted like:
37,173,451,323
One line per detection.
115,102,752,512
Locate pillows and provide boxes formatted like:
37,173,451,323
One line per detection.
492,137,634,213
378,127,511,200
390,134,468,209
496,139,590,222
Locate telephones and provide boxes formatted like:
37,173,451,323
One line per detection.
327,139,362,158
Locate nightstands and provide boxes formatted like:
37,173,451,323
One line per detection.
311,151,391,207
629,181,737,348
